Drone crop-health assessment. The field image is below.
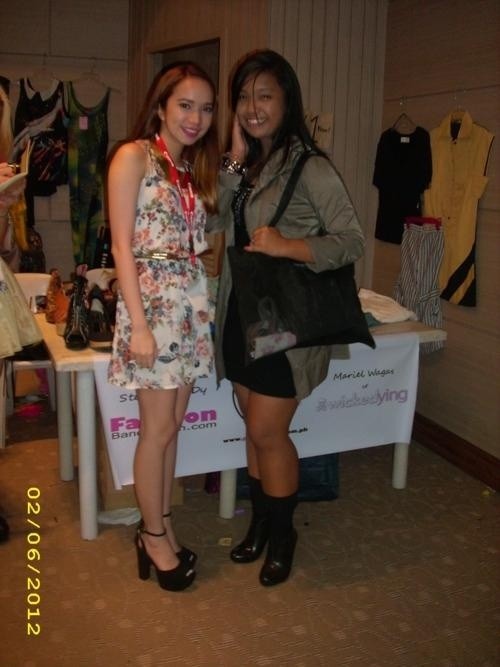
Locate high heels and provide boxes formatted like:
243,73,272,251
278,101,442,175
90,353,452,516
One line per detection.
135,512,196,591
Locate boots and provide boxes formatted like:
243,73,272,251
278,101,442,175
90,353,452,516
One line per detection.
231,475,269,562
260,491,297,586
46,264,117,349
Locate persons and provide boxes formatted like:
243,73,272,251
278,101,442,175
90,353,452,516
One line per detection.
106,61,224,591
212,47,367,587
0,86,45,543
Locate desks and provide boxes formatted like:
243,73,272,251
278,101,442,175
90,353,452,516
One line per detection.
34,313,449,542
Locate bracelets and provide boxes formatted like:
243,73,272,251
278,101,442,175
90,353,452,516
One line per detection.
220,152,246,176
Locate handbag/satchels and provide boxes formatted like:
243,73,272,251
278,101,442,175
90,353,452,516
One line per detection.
226,247,375,366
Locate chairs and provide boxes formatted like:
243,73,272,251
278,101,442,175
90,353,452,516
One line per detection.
0,273,55,415
86,265,115,289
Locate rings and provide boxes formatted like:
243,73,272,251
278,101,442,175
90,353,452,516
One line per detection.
252,241,256,245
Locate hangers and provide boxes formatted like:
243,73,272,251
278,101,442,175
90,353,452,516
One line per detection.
405,198,442,230
28,53,57,81
72,56,122,93
391,95,417,133
451,88,467,122
